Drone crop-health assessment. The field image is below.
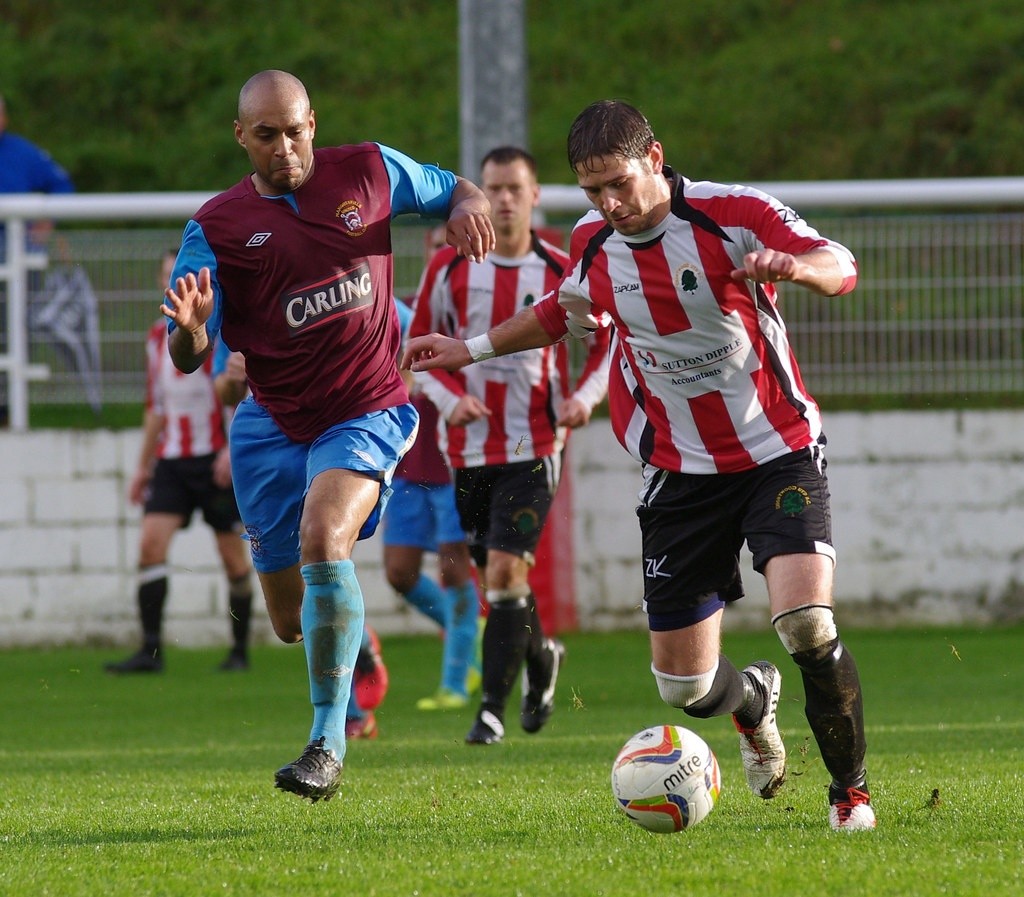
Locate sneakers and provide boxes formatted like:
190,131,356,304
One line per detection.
102,648,166,677
519,636,566,734
345,715,378,740
462,616,488,697
465,703,506,744
272,738,342,804
732,661,787,798
355,622,387,712
413,686,474,716
216,651,249,670
829,779,876,834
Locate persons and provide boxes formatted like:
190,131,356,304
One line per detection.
104,245,258,676
383,219,492,711
1,100,76,421
157,66,499,807
404,143,616,748
208,293,424,741
399,97,881,833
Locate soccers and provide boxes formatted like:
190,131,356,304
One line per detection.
611,725,722,833
